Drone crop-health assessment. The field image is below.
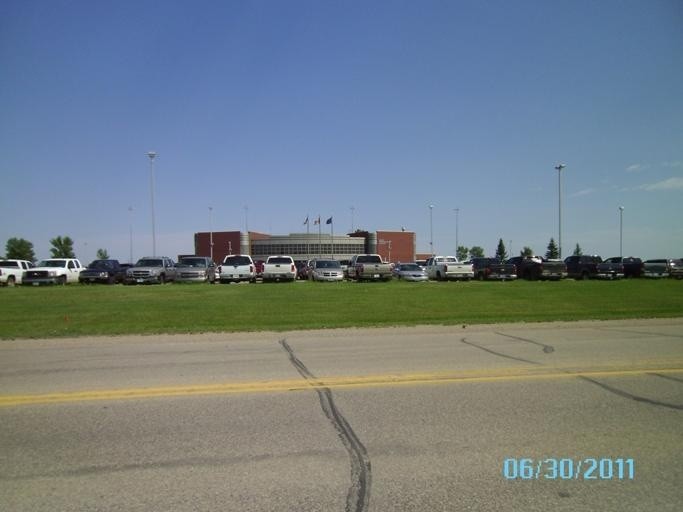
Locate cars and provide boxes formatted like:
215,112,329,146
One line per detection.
297,266,308,280
338,258,351,277
392,262,428,282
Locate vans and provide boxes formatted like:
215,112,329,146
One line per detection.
308,257,345,282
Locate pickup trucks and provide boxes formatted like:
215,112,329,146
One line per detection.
0,253,297,286
413,255,683,281
347,254,394,281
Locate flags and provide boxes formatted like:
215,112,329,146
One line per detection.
303,217,307,224
326,217,332,225
314,217,319,225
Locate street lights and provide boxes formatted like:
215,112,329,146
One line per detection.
554,163,566,260
144,151,159,257
349,206,356,232
242,205,250,230
429,205,435,254
619,205,624,255
452,207,460,254
207,205,215,261
128,206,134,264
